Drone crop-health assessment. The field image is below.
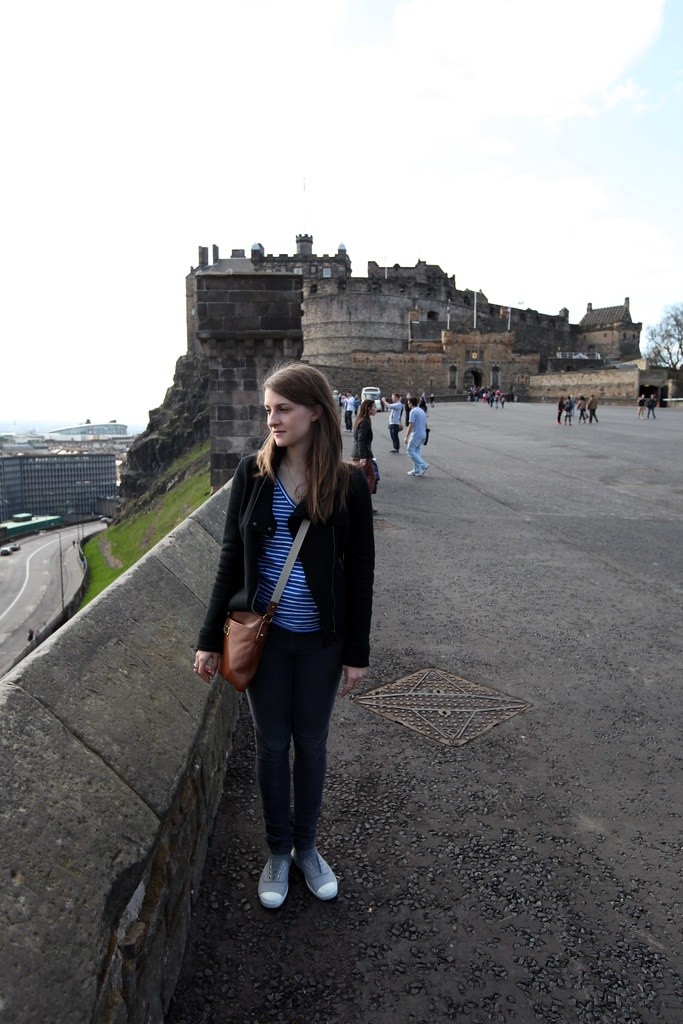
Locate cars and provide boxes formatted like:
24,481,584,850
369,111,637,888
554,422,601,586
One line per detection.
1,547,12,555
10,542,20,552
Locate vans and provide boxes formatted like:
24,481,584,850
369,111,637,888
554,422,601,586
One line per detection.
361,386,382,412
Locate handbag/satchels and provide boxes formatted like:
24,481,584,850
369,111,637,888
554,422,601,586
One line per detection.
398,423,403,432
220,611,272,691
369,458,380,494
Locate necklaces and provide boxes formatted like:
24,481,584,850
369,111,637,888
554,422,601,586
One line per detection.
283,455,311,502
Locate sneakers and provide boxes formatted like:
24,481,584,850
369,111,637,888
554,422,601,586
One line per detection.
343,428,348,431
416,464,429,476
346,430,352,433
407,469,420,477
259,852,293,908
290,845,337,901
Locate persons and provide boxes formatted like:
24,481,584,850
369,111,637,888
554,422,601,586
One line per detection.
351,398,380,516
190,363,375,912
646,394,660,419
26,627,41,646
72,540,76,548
380,390,405,453
403,396,431,477
635,394,646,421
341,391,355,433
332,385,599,428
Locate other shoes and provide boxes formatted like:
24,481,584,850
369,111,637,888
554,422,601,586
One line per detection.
372,508,377,513
389,449,398,454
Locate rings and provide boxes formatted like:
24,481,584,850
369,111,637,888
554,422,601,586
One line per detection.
346,684,353,688
192,663,198,669
